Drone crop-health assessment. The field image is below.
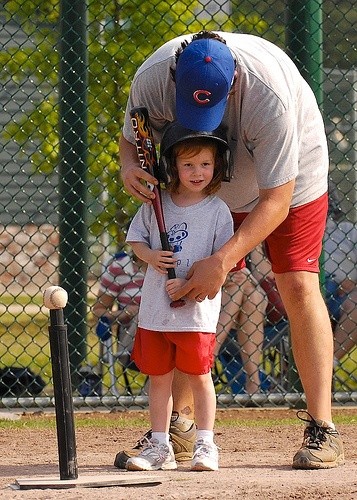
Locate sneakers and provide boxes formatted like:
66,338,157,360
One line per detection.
126,437,177,471
191,436,222,470
293,410,345,468
114,411,196,468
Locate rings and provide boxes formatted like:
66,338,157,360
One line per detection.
198,296,203,301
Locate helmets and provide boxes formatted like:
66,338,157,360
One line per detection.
160,116,234,187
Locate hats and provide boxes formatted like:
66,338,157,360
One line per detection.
175,38,235,132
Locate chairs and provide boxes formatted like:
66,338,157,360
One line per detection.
97,317,131,397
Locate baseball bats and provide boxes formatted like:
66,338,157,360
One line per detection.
129,105,187,309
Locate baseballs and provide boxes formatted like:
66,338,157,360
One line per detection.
42,286,69,310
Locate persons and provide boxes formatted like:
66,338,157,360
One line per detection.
111,30,348,470
92,126,356,472
